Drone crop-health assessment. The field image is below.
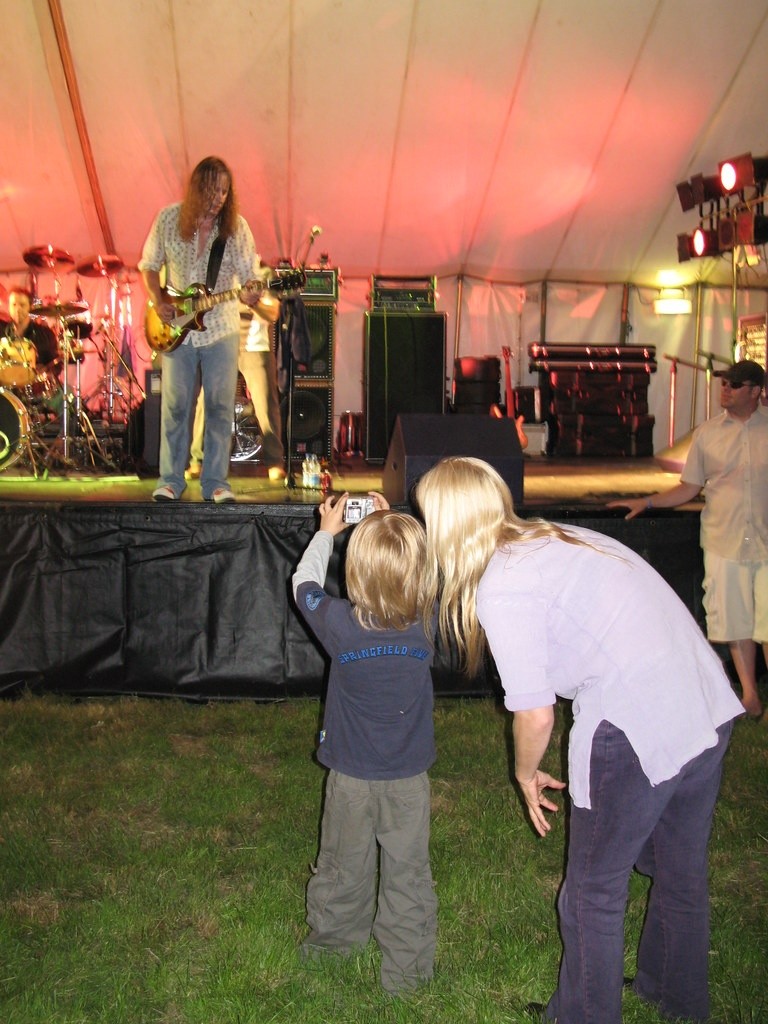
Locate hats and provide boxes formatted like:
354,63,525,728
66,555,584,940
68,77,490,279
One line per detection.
713,360,765,387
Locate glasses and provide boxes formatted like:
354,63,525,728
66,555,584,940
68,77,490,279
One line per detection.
720,379,753,389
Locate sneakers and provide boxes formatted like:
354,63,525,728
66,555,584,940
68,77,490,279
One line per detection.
203,486,237,503
152,486,184,501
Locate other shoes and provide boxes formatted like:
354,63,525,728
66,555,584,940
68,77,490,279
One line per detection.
521,1002,551,1024
732,701,766,722
622,976,642,998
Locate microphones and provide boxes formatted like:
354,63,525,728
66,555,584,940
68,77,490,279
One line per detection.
312,225,323,236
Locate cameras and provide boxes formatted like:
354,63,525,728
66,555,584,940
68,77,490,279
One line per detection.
342,496,376,524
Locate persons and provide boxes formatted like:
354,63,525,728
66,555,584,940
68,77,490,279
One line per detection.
606,360,768,723
137,155,287,508
415,453,748,1024
291,490,455,995
0,286,76,470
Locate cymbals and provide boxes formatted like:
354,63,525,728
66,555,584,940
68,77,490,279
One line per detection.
27,303,90,318
20,245,76,274
73,253,124,278
56,348,98,355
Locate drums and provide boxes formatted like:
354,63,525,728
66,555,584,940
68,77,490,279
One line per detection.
61,319,93,339
0,386,29,472
14,370,61,404
0,336,38,388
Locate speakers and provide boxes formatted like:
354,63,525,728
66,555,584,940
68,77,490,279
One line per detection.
143,369,161,472
274,300,336,382
454,356,501,413
382,413,524,507
277,381,335,465
365,310,447,463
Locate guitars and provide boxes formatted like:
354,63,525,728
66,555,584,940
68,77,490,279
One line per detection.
143,269,308,354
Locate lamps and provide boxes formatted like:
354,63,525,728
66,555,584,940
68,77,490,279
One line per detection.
675,151,768,263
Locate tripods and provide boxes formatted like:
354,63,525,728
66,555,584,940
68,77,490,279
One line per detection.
237,234,363,501
36,271,142,481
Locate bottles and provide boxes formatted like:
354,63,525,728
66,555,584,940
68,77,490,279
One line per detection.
302,454,332,497
